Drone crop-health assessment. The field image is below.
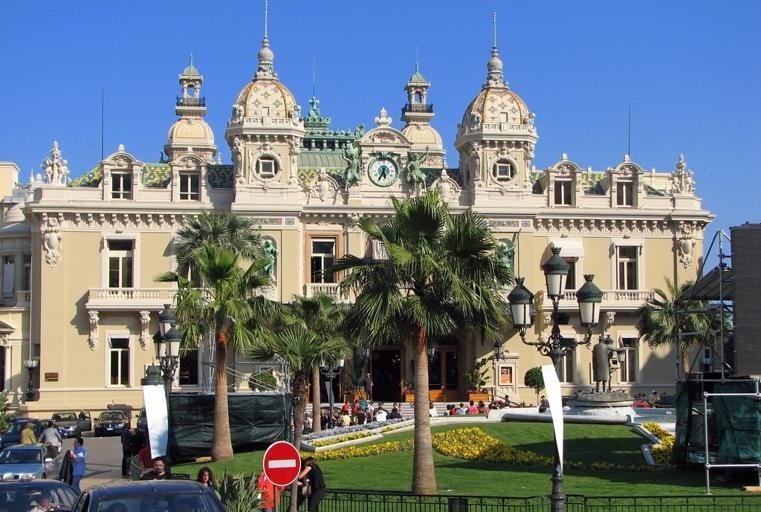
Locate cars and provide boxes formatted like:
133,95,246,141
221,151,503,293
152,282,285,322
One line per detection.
94,410,130,436
0,418,61,450
0,479,80,511
72,480,229,512
0,442,64,482
49,411,92,438
136,409,148,432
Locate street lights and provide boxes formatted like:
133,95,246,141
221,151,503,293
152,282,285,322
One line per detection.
507,247,604,511
152,303,183,447
319,357,345,429
605,335,626,392
22,358,40,401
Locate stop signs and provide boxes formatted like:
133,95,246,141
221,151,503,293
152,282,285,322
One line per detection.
263,440,302,487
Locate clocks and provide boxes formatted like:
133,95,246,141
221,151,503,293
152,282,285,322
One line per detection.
368,152,402,186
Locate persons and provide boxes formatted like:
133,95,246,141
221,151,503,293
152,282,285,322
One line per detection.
196,467,212,487
540,396,549,407
634,390,667,406
69,437,87,492
406,144,428,188
303,399,403,430
429,395,510,417
287,456,326,511
121,423,144,475
140,458,170,480
21,421,62,458
340,144,360,192
